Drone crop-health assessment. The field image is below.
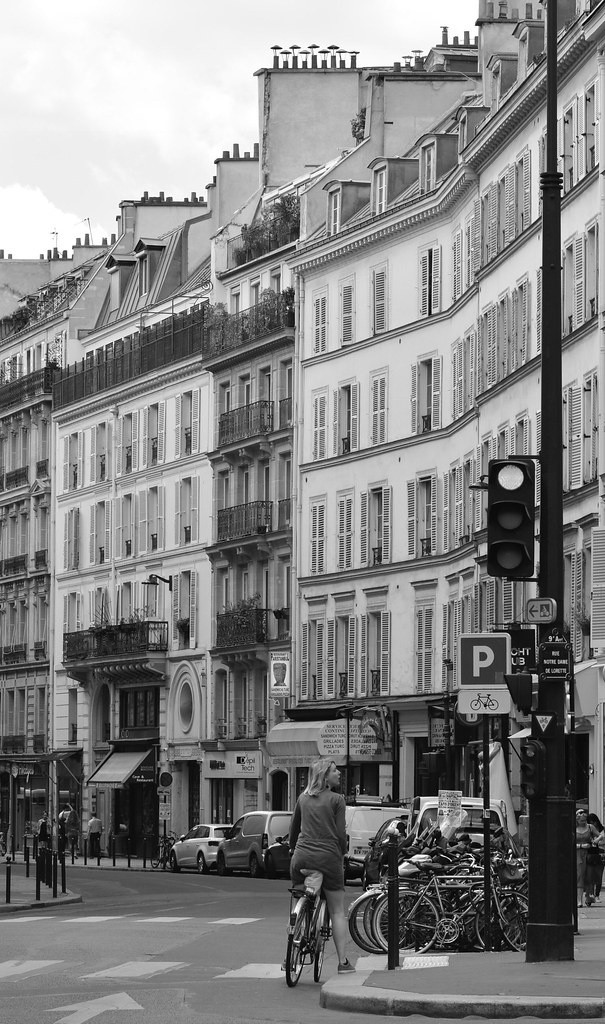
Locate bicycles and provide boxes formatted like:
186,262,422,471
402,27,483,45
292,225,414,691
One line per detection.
0,832,7,856
285,870,331,988
347,827,529,953
151,836,175,871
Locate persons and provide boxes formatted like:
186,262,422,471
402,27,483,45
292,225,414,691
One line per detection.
87,811,103,859
575,809,602,907
59,803,74,852
36,810,52,849
281,759,356,974
585,813,605,902
66,810,81,859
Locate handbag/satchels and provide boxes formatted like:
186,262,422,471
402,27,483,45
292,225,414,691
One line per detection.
587,846,602,865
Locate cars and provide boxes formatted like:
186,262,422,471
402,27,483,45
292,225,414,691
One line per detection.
264,833,291,880
361,817,408,891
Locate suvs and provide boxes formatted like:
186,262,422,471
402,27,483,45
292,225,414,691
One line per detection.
169,823,234,874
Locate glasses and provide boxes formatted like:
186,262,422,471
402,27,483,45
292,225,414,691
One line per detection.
577,811,587,815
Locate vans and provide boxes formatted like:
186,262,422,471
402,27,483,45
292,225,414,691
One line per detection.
216,811,294,878
345,805,410,879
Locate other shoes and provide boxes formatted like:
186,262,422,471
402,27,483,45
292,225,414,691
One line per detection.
281,961,294,971
337,958,356,973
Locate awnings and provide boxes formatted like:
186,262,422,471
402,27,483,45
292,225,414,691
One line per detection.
85,746,158,788
0,746,84,785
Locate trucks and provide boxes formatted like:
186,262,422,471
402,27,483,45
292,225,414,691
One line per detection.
408,796,506,850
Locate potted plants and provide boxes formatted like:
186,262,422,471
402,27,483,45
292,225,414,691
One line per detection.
216,591,268,647
203,289,295,356
443,659,453,671
65,600,159,660
576,614,590,637
42,361,59,393
234,191,299,266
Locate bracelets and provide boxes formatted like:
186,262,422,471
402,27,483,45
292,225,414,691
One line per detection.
580,844,582,848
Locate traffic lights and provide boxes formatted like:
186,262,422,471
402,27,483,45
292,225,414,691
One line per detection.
504,673,533,715
486,458,535,579
